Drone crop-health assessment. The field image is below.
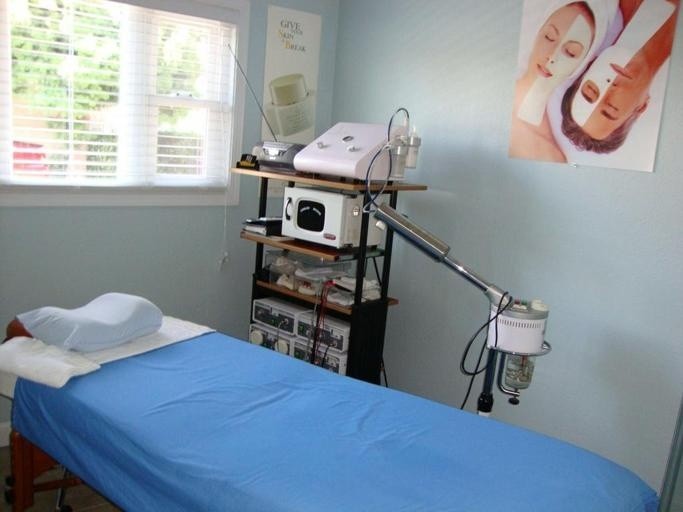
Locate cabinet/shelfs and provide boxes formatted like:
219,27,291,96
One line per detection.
224,168,428,385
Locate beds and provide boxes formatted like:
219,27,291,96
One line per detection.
0,316,660,512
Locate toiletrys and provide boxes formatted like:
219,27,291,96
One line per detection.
264,74,315,137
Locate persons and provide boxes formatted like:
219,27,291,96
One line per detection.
561,0,680,154
509,1,595,163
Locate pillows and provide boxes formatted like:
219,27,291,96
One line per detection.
15,291,165,352
